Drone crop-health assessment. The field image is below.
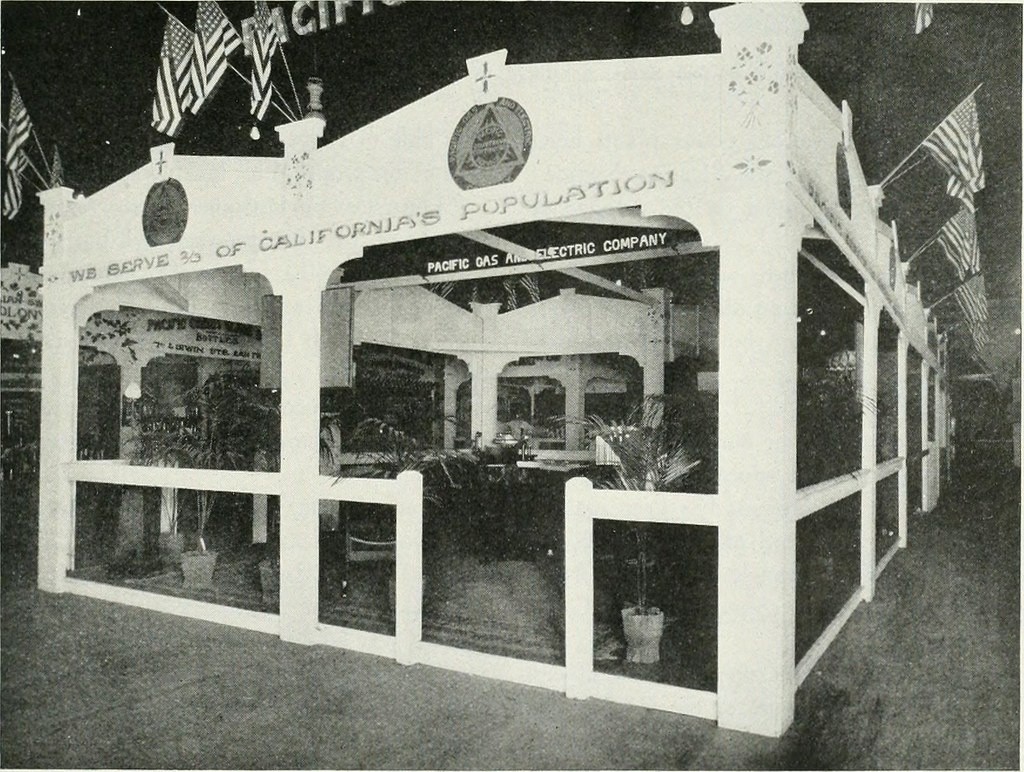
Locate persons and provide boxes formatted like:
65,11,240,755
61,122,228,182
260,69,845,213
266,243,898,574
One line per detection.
497,409,545,441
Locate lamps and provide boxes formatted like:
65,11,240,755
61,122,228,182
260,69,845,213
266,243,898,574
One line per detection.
301,76,327,138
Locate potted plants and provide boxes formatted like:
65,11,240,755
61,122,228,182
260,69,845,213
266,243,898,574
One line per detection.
119,374,460,590
546,392,703,665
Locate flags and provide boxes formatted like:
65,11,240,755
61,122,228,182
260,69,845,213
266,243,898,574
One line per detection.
954,274,989,327
188,2,243,119
964,315,993,352
914,3,933,34
920,95,986,212
251,1,279,120
152,17,194,137
3,150,30,220
6,83,34,171
937,208,982,281
